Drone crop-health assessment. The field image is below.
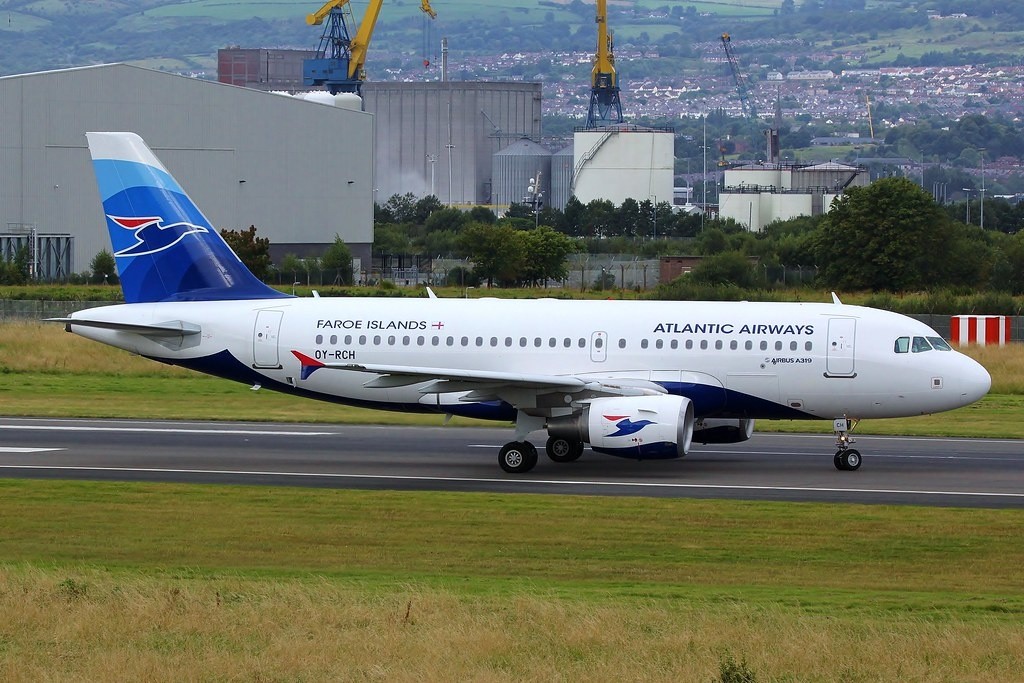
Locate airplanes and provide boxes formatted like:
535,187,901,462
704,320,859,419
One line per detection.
39,131,993,475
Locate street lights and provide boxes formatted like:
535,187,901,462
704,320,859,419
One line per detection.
920,150,925,192
536,191,543,228
650,195,657,237
933,182,948,203
977,148,988,199
426,154,440,216
799,149,803,164
854,147,860,169
293,282,300,297
445,145,456,209
978,189,988,229
962,188,971,225
